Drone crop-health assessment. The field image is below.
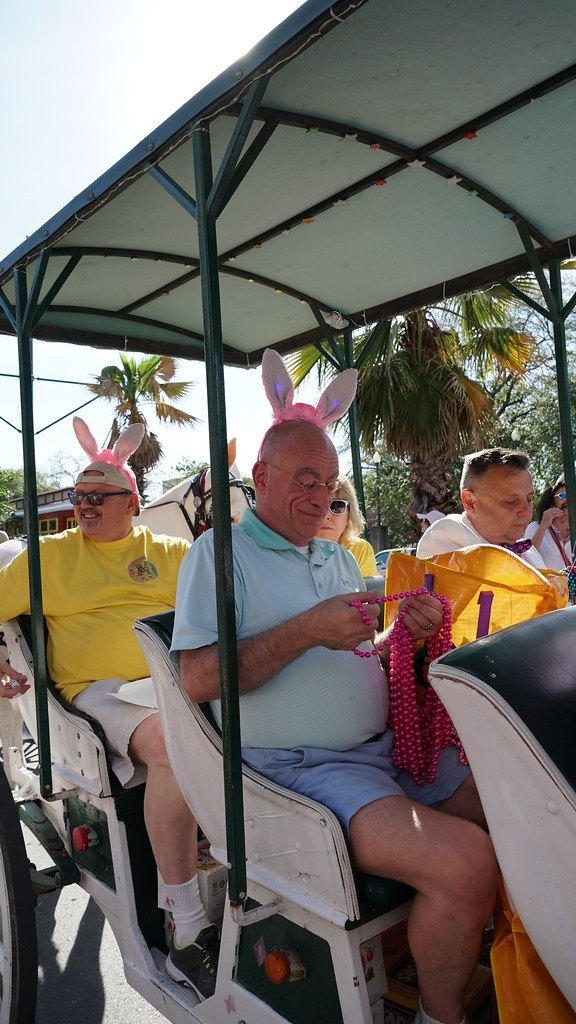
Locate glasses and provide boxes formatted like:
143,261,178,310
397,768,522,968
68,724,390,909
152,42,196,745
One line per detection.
266,462,341,495
328,499,353,516
67,490,132,507
554,493,568,500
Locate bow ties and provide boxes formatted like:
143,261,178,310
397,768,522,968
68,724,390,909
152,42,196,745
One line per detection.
499,538,533,555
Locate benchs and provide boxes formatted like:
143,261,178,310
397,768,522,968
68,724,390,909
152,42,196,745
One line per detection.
0,619,112,801
134,611,416,932
426,605,576,1012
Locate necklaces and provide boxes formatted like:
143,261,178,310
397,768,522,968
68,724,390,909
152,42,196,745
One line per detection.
553,527,571,542
350,588,469,786
559,564,576,602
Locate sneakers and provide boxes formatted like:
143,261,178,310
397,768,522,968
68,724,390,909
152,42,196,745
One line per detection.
164,923,222,1005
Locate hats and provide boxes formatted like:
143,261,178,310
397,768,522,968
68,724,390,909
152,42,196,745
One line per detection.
416,510,446,524
74,460,141,519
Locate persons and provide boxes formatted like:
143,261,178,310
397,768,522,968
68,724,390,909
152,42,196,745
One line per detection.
166,419,497,1024
523,473,572,573
416,510,446,534
314,476,379,578
416,449,576,607
0,461,219,1004
